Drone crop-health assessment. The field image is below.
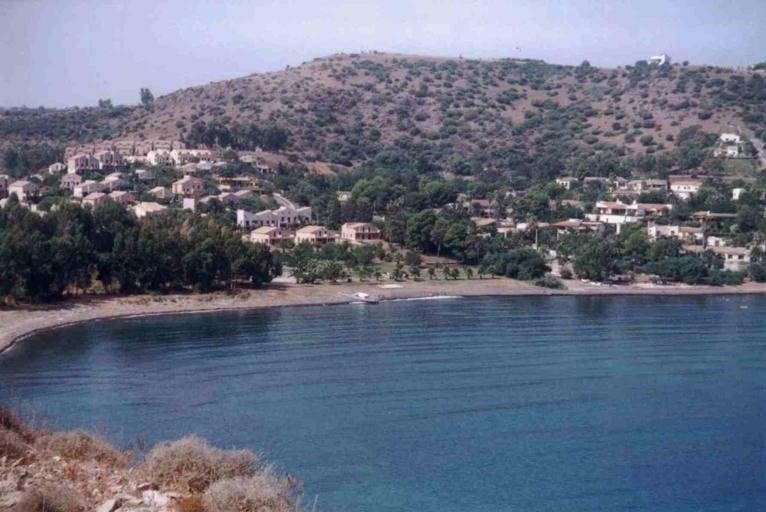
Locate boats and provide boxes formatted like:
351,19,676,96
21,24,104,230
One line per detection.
335,290,378,303
